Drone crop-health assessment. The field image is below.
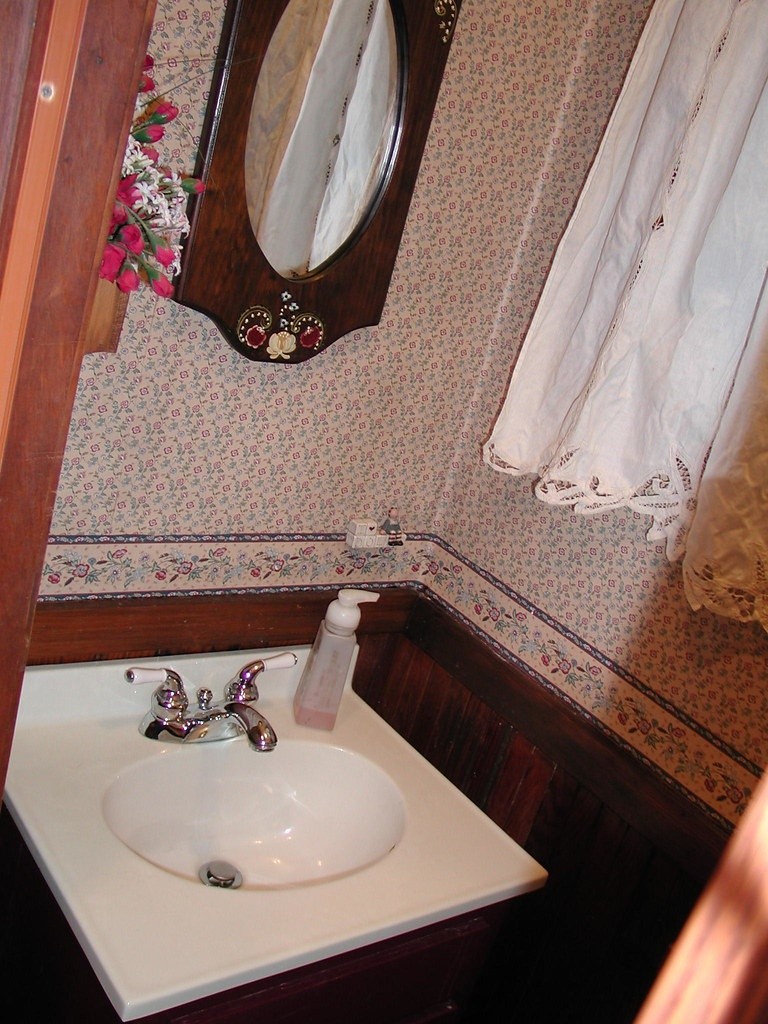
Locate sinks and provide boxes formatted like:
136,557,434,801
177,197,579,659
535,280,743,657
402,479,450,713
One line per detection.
100,735,409,893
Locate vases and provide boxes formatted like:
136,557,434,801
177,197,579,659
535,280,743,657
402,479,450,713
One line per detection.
86,276,132,354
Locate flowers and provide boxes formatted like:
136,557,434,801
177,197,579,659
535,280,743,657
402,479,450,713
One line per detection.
102,53,213,300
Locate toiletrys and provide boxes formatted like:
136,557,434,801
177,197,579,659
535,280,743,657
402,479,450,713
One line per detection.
293,589,382,732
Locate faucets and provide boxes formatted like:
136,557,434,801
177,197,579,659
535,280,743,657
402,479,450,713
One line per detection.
124,650,298,752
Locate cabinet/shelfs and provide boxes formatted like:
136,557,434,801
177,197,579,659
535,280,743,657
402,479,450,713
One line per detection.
0,798,511,1024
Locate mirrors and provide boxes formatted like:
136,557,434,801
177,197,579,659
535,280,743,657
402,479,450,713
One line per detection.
172,0,463,364
